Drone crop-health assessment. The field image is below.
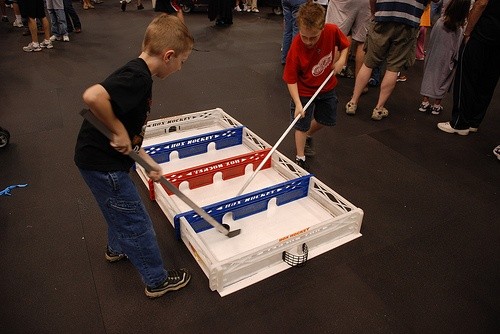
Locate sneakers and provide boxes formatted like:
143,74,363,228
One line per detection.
371,108,388,119
105,246,125,262
50,35,62,41
437,122,469,135
345,102,358,113
432,105,443,114
63,35,70,41
13,19,23,27
145,268,191,297
304,137,315,155
295,156,306,171
23,42,42,51
419,103,432,112
40,39,54,48
469,127,478,132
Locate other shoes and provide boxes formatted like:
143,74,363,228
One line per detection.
120,0,131,4
39,30,45,33
182,4,190,12
75,29,81,33
234,6,242,11
273,6,282,15
137,3,144,9
252,7,259,12
83,4,88,9
121,1,127,11
22,27,30,35
87,3,95,8
336,51,425,94
94,0,104,3
243,7,251,12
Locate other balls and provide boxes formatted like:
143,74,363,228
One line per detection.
220,223,230,233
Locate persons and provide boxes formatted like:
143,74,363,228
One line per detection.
345,0,431,120
437,0,500,135
74,14,194,297
281,0,311,64
0,0,145,51
208,0,259,25
283,2,351,169
326,0,382,85
396,0,474,115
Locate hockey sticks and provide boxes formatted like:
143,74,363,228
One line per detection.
79,107,242,239
235,69,334,197
